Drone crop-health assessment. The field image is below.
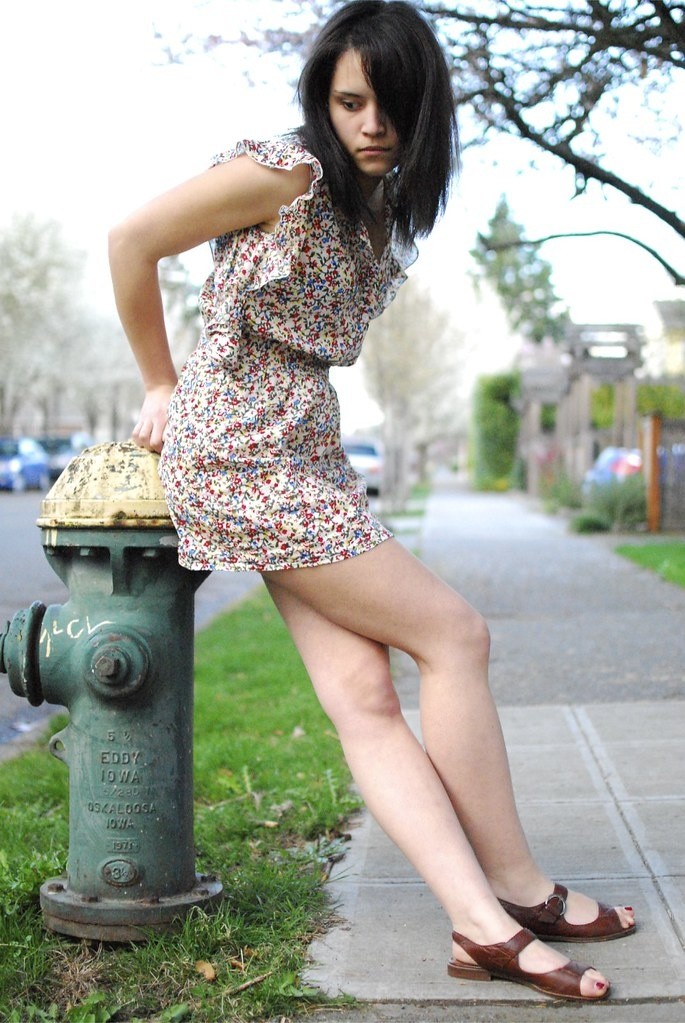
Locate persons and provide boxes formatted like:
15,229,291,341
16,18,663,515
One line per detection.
107,0,637,1001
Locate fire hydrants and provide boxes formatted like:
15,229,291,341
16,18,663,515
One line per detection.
1,435,228,948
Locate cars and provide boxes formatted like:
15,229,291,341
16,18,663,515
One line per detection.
343,441,382,496
1,437,50,492
584,447,685,507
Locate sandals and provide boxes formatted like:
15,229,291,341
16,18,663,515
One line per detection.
496,883,636,943
447,928,611,1001
